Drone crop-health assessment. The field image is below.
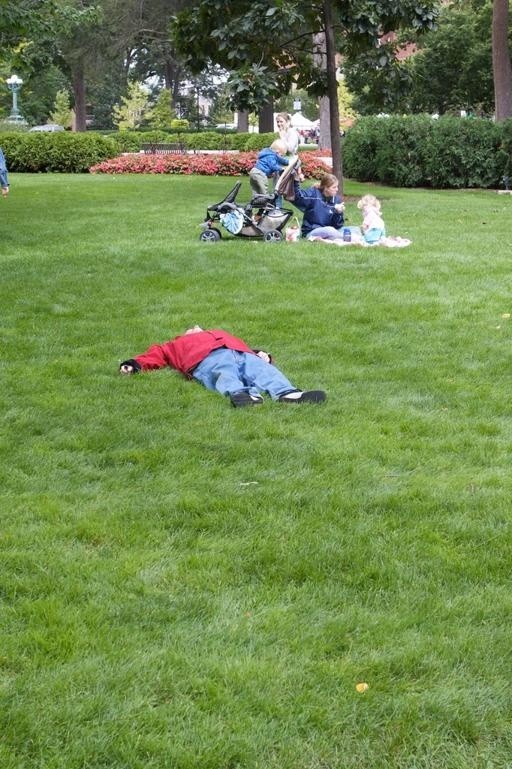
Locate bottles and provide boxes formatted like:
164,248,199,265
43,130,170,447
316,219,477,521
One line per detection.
343,228,351,242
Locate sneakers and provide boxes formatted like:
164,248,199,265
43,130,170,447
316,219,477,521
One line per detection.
279,390,327,405
231,393,263,407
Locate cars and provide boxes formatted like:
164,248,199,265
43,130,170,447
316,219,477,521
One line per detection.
29,124,64,132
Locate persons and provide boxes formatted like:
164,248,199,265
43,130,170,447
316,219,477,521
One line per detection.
339,130,346,137
249,138,289,201
357,193,385,241
287,172,346,240
118,325,327,409
273,111,306,210
0,148,10,200
296,127,320,145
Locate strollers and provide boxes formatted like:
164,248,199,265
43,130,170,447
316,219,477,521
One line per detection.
199,154,301,244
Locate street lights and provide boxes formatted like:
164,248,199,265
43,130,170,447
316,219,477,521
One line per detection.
6,75,24,120
